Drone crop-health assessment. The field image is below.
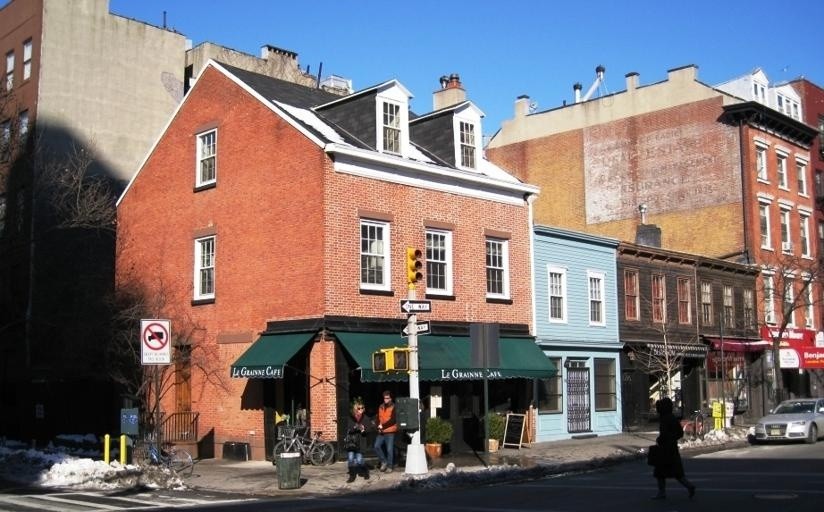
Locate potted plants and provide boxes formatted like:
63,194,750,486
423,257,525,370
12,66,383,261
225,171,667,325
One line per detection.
483,412,505,453
425,417,453,459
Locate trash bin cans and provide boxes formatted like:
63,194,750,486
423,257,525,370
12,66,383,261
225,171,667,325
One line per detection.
275,455,301,490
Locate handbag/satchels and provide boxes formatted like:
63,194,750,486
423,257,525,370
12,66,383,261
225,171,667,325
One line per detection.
648,445,658,466
343,434,360,452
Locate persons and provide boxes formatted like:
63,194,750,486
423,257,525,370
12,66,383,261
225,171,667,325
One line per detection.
342,399,376,483
374,390,397,472
649,398,696,496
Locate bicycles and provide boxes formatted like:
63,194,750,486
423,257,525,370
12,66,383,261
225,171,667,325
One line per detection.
273,414,335,466
682,406,713,442
138,440,194,479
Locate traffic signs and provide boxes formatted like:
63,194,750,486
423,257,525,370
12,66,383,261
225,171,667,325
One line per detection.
398,320,431,339
400,299,432,315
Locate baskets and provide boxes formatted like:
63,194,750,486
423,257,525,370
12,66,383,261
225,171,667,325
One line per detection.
280,427,292,437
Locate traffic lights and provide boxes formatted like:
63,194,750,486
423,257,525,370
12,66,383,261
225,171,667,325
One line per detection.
371,350,390,374
389,347,411,372
407,247,423,282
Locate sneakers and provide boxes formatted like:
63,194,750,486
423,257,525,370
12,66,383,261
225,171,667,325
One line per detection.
385,467,392,473
689,485,695,497
380,461,387,471
650,496,666,500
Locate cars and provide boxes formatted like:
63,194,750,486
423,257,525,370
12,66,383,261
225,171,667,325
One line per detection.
754,397,824,444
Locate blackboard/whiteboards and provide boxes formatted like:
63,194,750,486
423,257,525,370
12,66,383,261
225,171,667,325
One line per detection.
502,413,526,446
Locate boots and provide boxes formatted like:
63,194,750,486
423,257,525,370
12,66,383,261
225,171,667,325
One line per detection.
346,466,356,483
360,465,369,479
